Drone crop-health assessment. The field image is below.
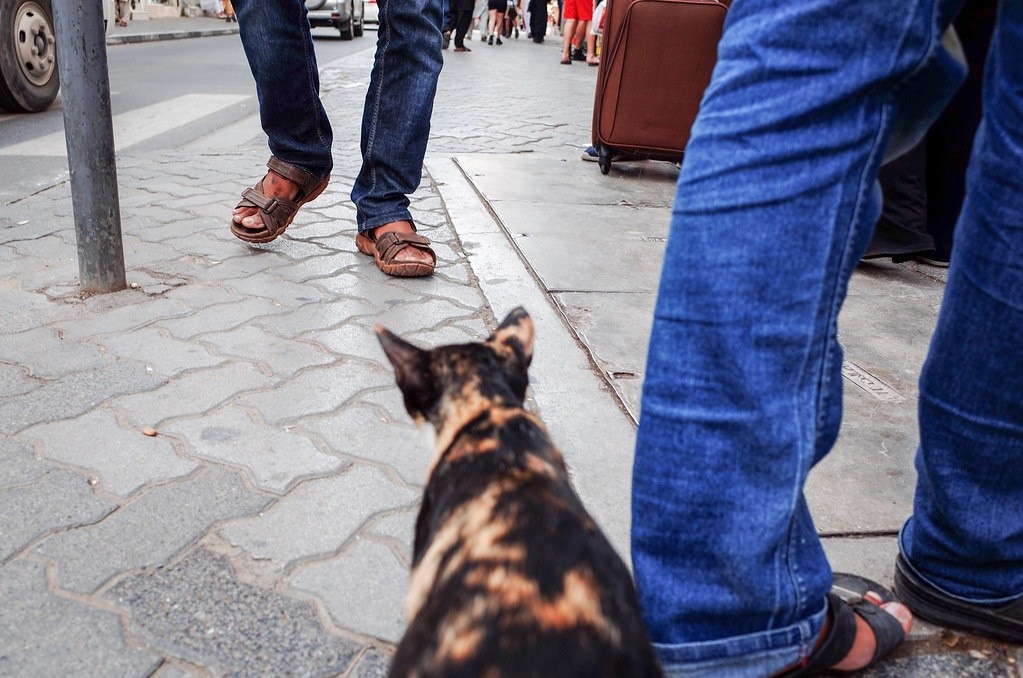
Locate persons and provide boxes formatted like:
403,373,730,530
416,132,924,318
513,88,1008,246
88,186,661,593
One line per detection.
631,0,1023,678
226,0,449,276
442,0,600,67
199,0,237,23
117,0,131,26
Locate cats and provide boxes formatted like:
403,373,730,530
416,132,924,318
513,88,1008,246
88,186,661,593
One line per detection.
372,305,665,678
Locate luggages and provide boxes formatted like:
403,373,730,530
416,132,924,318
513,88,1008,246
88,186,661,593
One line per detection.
592,0,728,176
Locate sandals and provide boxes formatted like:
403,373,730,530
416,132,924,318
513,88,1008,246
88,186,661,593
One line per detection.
230,155,331,243
355,219,437,278
893,546,1023,647
771,569,904,678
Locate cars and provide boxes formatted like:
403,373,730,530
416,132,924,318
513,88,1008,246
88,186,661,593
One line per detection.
304,0,378,40
0,0,60,115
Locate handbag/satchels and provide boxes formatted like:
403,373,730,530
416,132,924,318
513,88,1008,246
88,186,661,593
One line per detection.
508,7,517,21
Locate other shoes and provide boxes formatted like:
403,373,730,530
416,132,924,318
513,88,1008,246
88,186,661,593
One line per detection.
580,145,647,163
465,35,472,39
515,28,519,38
588,58,599,66
560,56,572,65
496,38,503,45
480,36,487,43
453,45,472,52
488,34,494,45
443,31,451,50
571,48,586,61
